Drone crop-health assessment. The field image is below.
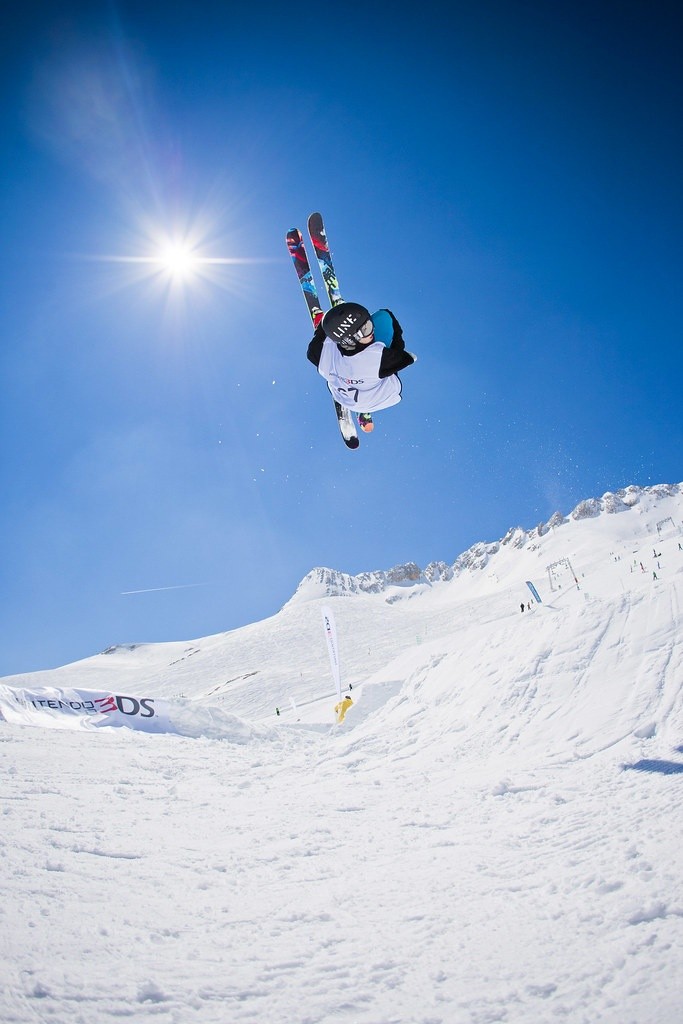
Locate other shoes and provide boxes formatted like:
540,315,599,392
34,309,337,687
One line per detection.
315,314,323,327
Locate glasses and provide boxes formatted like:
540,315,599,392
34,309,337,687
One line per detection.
337,317,374,344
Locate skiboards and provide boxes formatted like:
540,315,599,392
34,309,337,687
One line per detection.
285,225,362,451
306,210,376,436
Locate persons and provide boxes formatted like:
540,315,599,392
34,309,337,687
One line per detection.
306,298,418,413
335,695,353,722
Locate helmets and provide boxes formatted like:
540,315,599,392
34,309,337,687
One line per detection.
322,302,375,349
